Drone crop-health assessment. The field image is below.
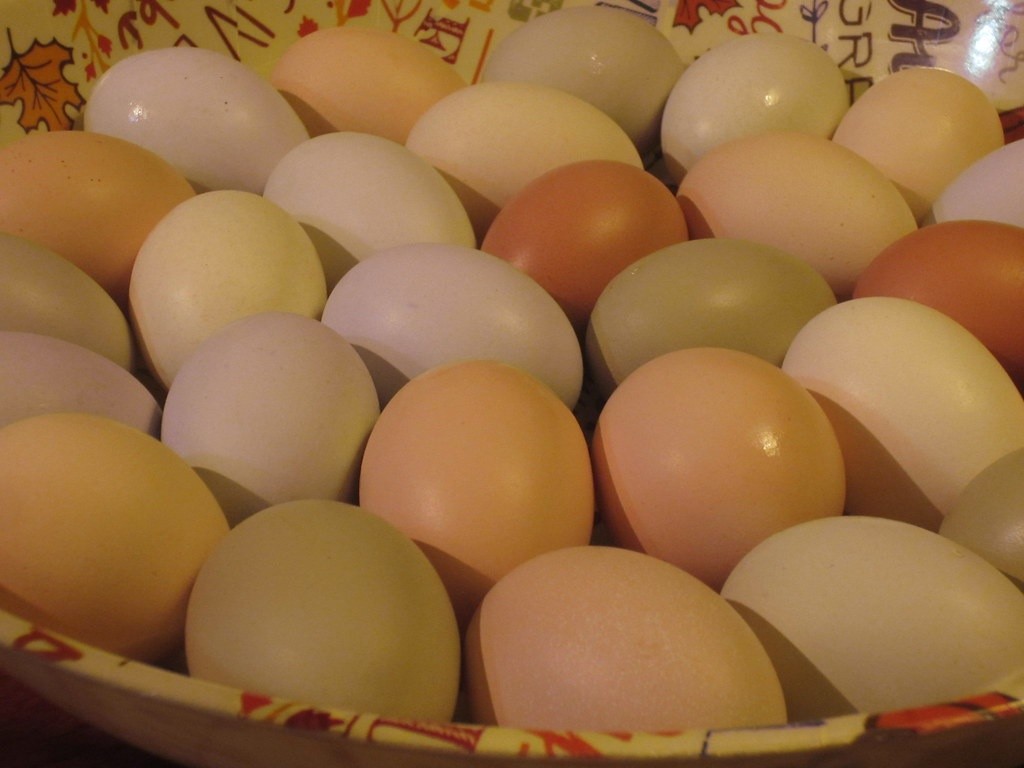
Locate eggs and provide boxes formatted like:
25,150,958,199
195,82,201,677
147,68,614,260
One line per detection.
0,7,1024,732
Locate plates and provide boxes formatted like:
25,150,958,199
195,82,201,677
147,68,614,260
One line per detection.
0,0,1024,768
